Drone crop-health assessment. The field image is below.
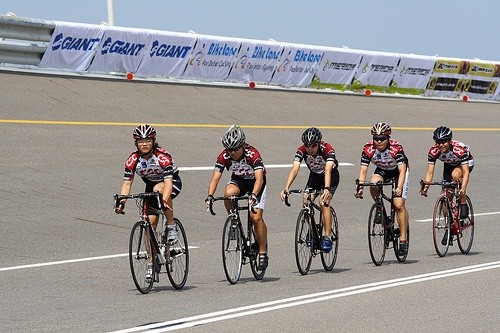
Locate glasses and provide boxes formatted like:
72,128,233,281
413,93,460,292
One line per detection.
304,144,317,148
374,137,386,142
226,148,240,152
435,140,448,144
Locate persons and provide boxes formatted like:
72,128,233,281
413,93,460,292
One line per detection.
280,127,340,250
419,127,475,245
114,125,182,280
354,121,411,253
205,125,267,270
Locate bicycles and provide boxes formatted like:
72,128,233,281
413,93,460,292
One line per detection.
285,188,339,277
355,178,409,267
419,179,474,258
113,191,189,296
206,194,268,284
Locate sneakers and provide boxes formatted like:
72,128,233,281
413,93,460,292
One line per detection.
166,223,180,245
144,263,154,279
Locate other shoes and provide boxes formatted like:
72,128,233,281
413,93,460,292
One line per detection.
374,206,384,224
442,230,453,245
256,252,269,270
322,238,333,251
460,203,469,219
398,240,408,256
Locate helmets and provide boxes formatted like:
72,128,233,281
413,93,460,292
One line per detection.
221,124,246,149
370,121,391,136
301,127,322,144
133,124,157,140
432,126,453,140
